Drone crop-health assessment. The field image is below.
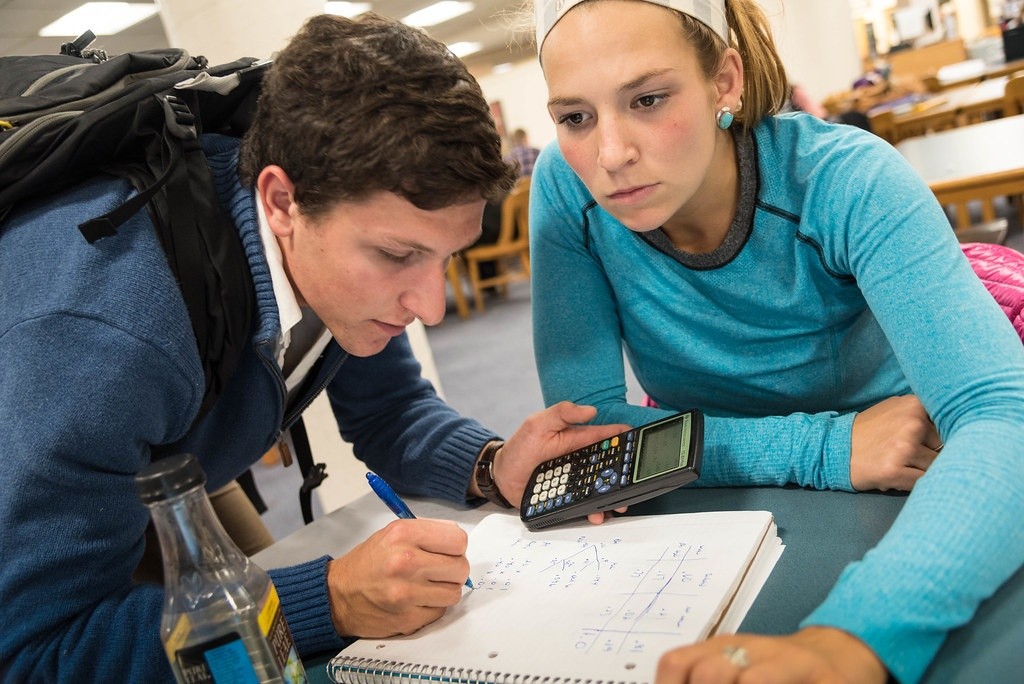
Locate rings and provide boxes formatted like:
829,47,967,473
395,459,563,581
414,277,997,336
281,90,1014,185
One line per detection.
720,646,751,670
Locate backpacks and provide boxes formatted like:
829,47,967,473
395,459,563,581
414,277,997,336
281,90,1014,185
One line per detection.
2,27,278,450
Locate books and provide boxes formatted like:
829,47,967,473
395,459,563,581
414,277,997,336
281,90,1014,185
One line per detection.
322,510,786,684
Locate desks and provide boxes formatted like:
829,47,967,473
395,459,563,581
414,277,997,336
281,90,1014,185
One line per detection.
242,486,1024,683
895,113,1024,229
868,69,1024,145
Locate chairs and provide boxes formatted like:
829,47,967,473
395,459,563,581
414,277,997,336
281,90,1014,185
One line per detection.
465,177,532,311
301,318,445,517
1006,71,1024,118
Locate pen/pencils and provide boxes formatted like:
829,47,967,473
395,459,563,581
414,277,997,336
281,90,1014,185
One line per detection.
365,471,475,591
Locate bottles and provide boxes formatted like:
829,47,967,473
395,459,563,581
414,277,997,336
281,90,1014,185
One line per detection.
131,451,309,684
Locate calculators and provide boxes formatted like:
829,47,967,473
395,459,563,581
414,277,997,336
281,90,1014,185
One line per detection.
521,407,704,533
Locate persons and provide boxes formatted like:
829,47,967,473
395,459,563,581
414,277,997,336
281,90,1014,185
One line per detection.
503,128,542,176
526,0,1024,683
452,201,519,295
1,11,635,684
